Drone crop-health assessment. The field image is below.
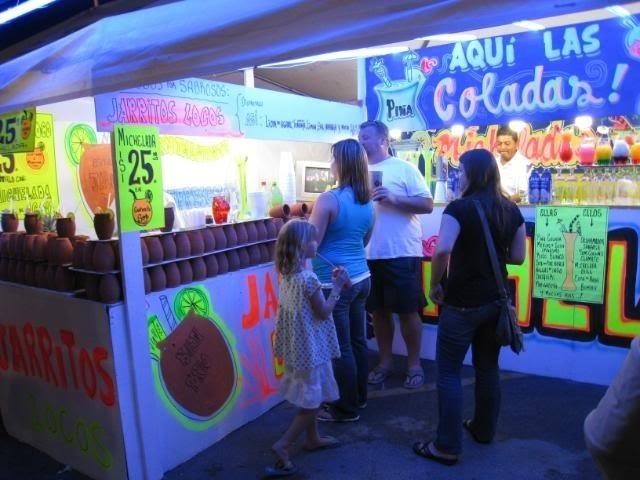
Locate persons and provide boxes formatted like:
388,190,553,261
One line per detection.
358,118,433,389
579,332,640,480
291,138,377,423
411,148,526,466
264,218,352,476
494,127,533,202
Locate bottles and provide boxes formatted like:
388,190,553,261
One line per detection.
260,182,283,217
212,191,238,223
527,169,640,204
559,129,640,165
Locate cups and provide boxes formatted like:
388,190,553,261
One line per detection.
140,215,309,293
160,206,175,232
0,232,124,302
332,268,352,291
397,150,434,192
270,202,291,218
94,213,115,240
1,214,19,231
56,217,76,237
23,213,43,233
389,140,422,170
290,203,308,216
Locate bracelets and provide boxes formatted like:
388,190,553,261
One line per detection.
329,292,341,301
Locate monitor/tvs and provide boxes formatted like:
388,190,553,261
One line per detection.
296,161,340,203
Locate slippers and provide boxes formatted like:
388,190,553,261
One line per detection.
465,417,490,445
367,362,401,384
304,435,340,451
413,440,457,465
316,401,368,422
266,459,297,475
403,367,424,389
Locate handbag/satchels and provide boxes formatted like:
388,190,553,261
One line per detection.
497,299,524,355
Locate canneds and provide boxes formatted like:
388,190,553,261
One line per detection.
333,266,352,289
